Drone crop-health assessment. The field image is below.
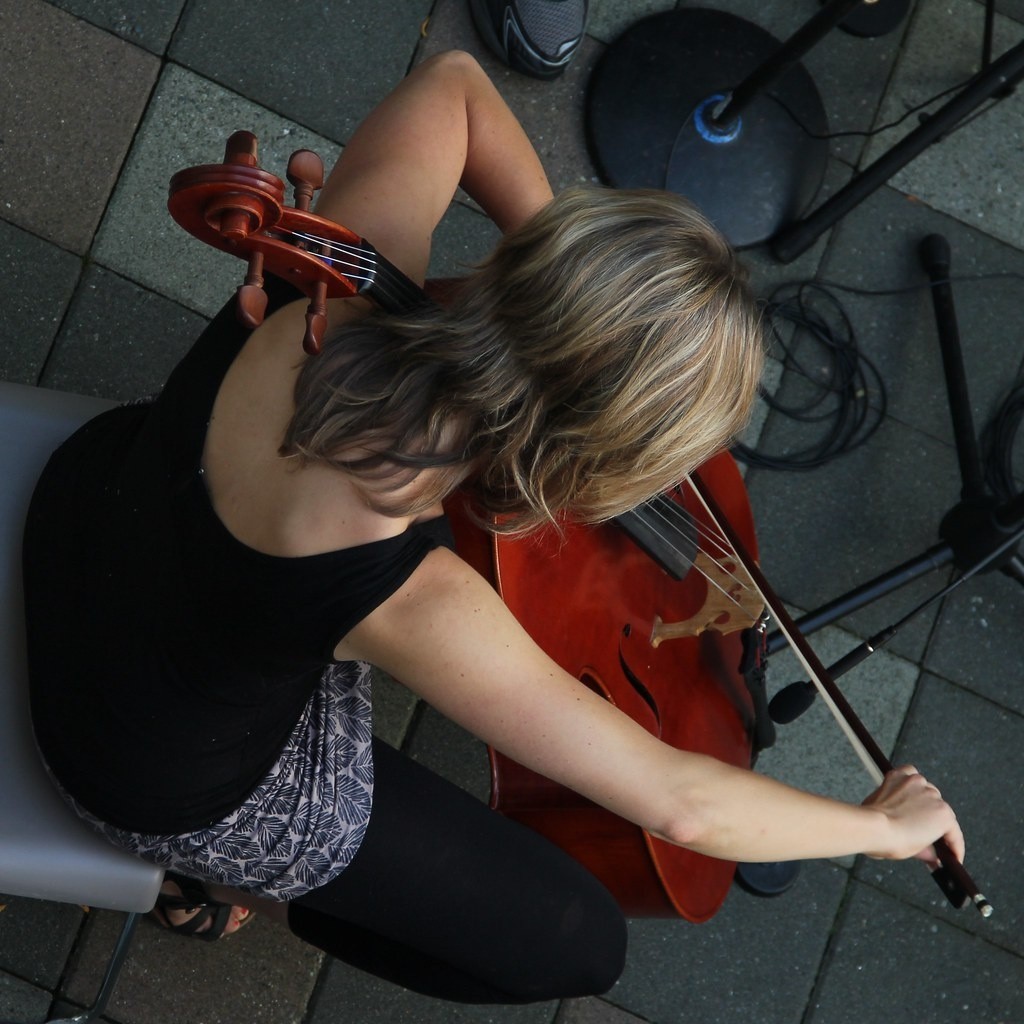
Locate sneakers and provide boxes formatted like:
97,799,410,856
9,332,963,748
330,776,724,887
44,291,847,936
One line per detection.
467,0,590,81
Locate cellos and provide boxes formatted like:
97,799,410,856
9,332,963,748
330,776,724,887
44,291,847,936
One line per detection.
169,128,773,922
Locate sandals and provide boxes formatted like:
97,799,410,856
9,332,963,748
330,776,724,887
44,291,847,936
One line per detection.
151,871,257,942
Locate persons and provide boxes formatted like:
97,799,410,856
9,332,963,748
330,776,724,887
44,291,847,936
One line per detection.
22,48,966,1005
